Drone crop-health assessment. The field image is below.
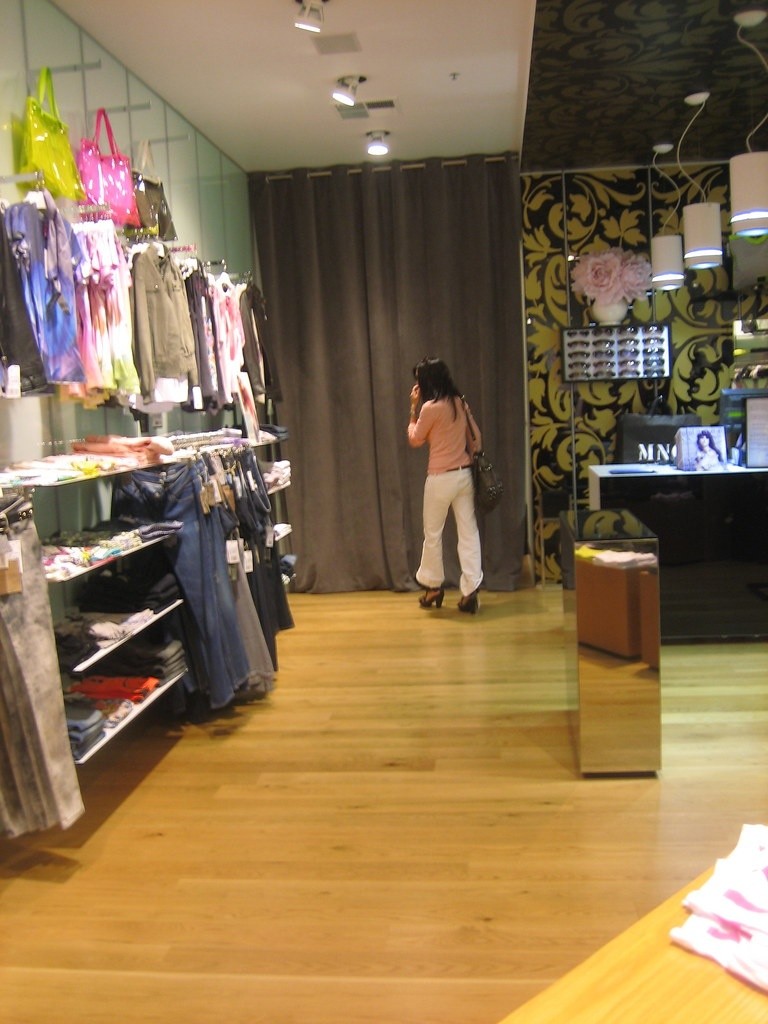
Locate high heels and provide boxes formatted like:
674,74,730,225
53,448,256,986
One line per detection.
458,593,478,615
419,587,444,608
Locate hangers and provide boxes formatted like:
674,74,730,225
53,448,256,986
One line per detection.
25,170,264,297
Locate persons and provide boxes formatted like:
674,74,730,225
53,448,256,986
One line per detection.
694,431,722,470
407,352,484,614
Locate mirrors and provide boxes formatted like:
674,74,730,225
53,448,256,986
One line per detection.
574,540,662,772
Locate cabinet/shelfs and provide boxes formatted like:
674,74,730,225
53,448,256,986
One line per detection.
0,424,297,767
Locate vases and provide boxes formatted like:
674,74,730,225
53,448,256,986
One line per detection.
591,297,627,325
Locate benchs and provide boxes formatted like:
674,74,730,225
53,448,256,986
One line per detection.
575,548,657,658
641,570,660,672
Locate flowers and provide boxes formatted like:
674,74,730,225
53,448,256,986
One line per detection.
571,248,653,305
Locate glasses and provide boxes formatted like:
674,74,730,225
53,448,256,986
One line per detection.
567,323,666,383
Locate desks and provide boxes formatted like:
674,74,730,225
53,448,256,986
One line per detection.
588,464,768,643
498,846,768,1024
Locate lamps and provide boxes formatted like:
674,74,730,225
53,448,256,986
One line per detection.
294,0,324,33
651,144,685,290
677,92,723,270
729,10,768,237
331,75,359,107
368,132,389,156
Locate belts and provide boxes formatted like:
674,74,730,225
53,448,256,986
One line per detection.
446,464,473,472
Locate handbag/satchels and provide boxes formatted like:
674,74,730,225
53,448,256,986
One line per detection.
116,139,177,244
73,108,142,229
11,65,88,202
474,449,504,517
616,396,701,465
